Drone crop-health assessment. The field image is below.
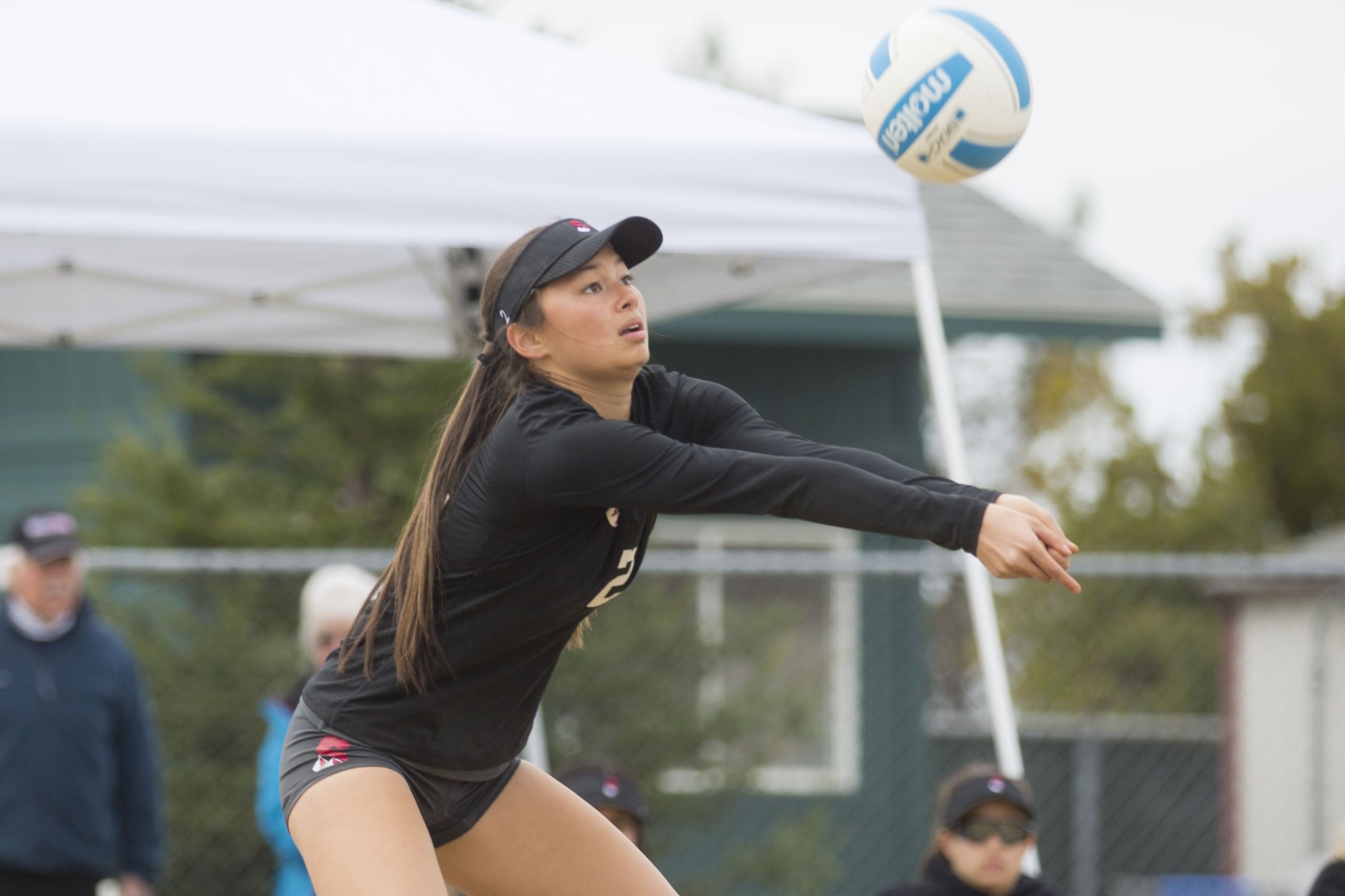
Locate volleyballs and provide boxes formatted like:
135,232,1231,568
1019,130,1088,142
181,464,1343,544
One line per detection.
856,7,1034,181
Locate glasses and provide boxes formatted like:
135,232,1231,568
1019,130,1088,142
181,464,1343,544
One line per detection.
956,818,1029,843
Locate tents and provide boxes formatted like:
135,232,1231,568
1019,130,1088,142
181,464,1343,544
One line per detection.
0,1,1160,882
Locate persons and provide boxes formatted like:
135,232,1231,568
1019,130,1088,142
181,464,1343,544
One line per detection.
857,757,1073,896
557,762,646,861
255,564,385,896
281,214,1080,896
0,505,174,896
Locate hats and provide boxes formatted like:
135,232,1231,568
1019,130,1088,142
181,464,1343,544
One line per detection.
941,774,1036,828
492,217,663,331
12,508,85,566
